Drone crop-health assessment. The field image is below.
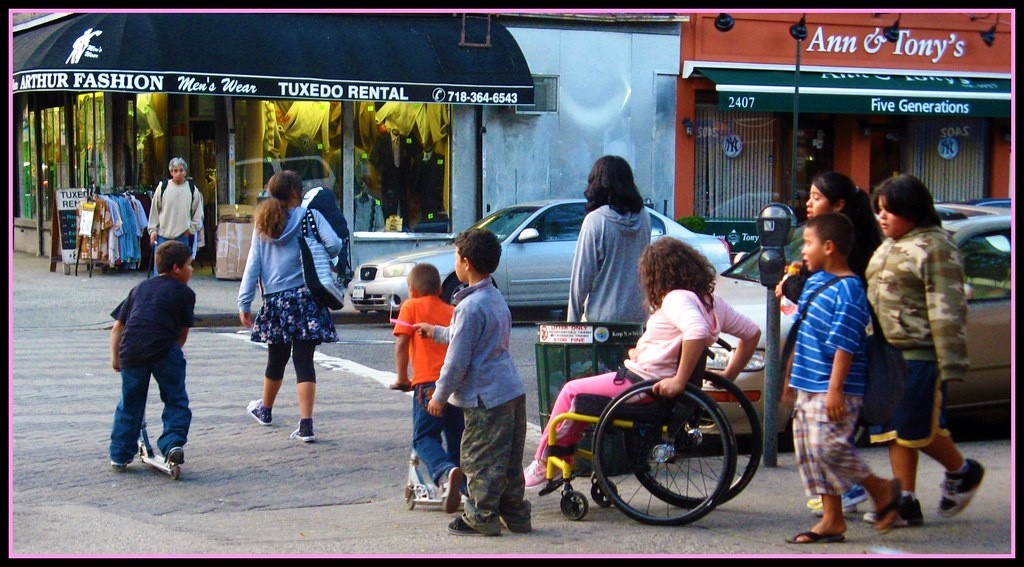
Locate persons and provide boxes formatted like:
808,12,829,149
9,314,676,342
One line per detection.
391,263,469,513
263,100,449,231
411,228,533,536
148,156,205,278
524,235,762,489
109,240,196,472
237,169,343,442
567,155,652,324
774,171,903,545
862,174,985,527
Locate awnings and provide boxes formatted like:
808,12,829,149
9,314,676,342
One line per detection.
13,13,535,105
692,61,1011,118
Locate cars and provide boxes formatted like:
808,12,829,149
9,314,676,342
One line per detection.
347,199,732,320
679,198,1011,469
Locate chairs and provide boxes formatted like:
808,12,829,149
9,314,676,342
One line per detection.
963,253,1010,300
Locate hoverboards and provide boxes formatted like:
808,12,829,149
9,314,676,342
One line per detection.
103,324,180,480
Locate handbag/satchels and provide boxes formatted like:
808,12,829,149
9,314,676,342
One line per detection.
865,332,914,433
298,209,345,311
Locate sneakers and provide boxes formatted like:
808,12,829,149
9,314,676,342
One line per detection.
111,460,126,471
446,513,485,536
290,418,315,442
863,494,924,527
936,458,985,518
806,484,868,516
438,466,462,514
164,446,184,465
247,399,273,426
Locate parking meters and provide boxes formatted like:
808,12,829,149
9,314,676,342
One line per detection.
757,202,797,468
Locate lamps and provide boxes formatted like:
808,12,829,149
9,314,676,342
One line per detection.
714,13,734,32
970,13,1000,47
789,13,808,41
873,13,901,42
683,118,692,137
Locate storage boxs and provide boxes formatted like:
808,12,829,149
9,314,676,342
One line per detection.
214,223,254,279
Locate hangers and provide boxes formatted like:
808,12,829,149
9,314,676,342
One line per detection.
83,184,147,201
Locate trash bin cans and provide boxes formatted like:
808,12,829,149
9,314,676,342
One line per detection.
535,321,645,476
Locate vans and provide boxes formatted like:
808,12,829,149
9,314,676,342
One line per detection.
235,154,336,204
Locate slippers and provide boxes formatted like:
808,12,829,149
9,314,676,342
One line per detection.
784,530,845,544
872,478,902,535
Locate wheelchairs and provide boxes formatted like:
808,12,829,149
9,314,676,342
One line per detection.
540,337,762,526
